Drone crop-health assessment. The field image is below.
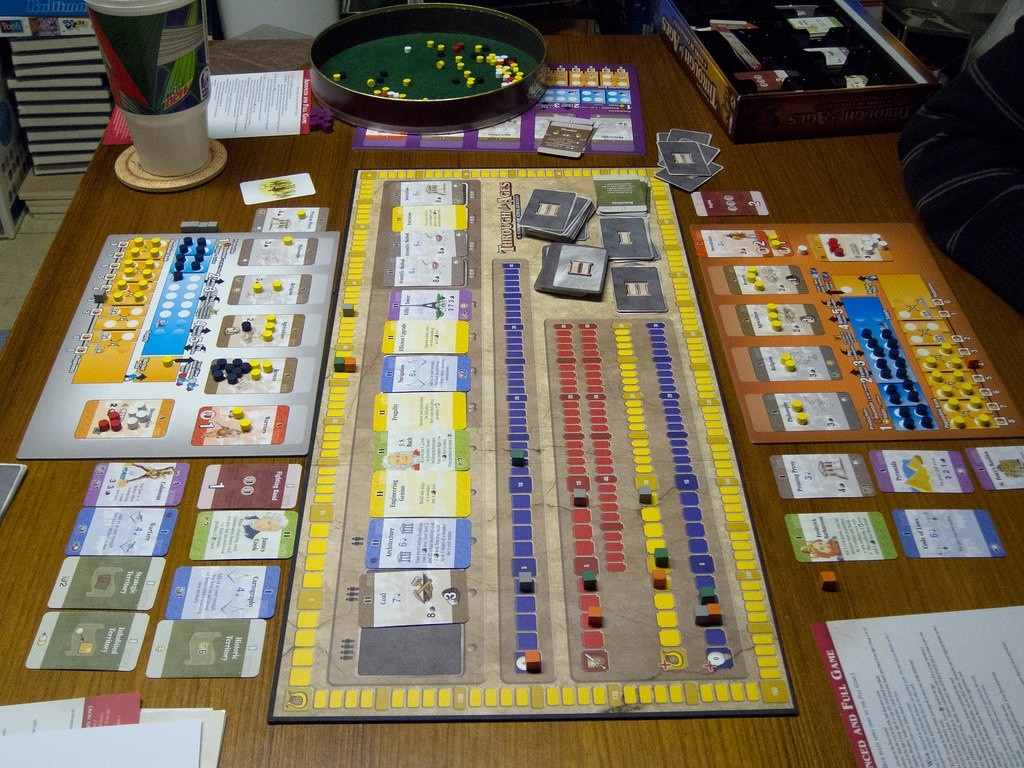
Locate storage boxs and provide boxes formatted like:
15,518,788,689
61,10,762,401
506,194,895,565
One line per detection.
652,0,942,145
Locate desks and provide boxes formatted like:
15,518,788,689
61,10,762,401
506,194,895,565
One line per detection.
1,40,1024,767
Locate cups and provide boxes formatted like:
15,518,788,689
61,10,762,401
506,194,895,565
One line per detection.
85,0,212,176
